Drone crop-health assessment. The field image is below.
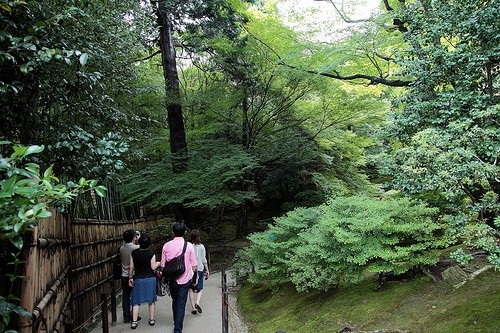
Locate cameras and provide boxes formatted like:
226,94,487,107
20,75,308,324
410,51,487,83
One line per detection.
136,231,140,238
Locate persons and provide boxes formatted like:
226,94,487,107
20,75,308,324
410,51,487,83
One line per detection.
161,223,198,333
187,230,209,314
120,229,141,323
129,234,161,329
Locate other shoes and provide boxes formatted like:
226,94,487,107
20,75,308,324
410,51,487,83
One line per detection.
191,311,197,314
131,321,138,329
131,316,141,322
195,304,202,313
149,319,155,325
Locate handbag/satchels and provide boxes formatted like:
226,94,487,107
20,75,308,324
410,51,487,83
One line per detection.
155,268,166,296
113,244,122,280
162,254,185,280
